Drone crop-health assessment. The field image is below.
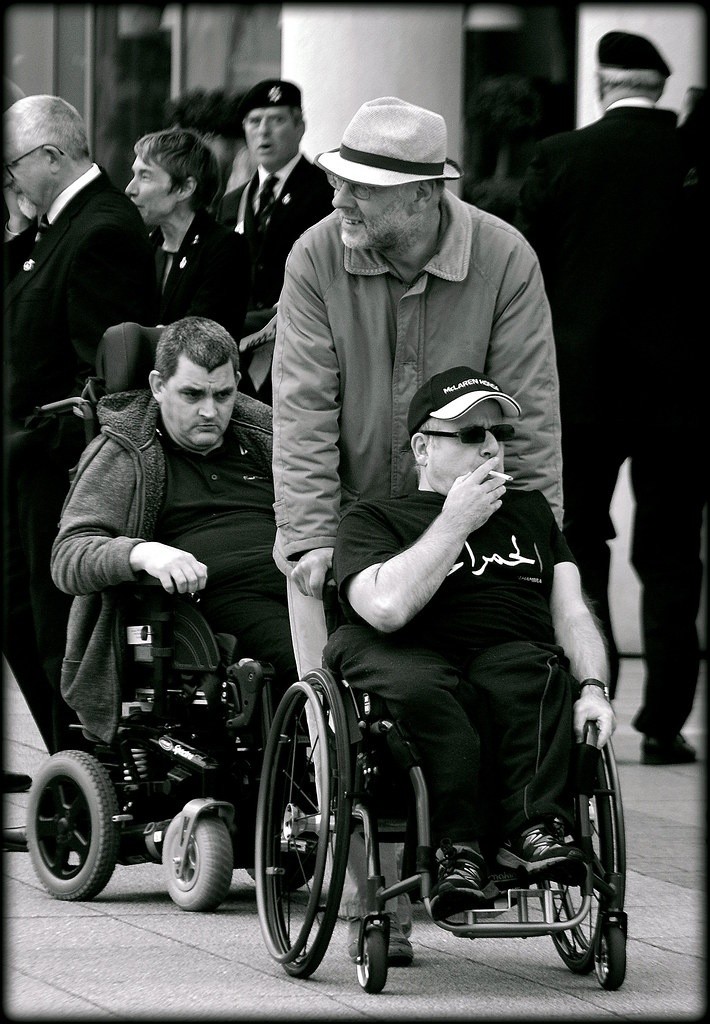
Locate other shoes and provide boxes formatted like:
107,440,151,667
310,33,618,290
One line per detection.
641,732,697,766
346,892,414,966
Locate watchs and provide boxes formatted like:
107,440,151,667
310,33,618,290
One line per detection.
577,678,611,703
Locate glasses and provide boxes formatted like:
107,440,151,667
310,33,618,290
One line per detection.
416,424,516,444
0,143,64,190
326,172,372,200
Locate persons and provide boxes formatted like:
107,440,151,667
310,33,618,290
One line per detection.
3,79,333,852
521,30,710,765
323,366,616,922
272,97,565,965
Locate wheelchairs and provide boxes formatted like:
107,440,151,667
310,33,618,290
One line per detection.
254,568,630,994
22,575,312,916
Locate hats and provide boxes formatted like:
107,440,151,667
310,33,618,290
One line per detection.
313,96,466,187
407,366,522,436
597,31,671,78
237,78,301,122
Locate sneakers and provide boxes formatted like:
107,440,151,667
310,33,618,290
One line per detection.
496,814,590,888
428,837,501,922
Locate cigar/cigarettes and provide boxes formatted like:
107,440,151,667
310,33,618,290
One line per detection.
489,470,514,481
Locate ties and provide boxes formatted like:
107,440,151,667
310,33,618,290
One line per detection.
254,176,279,232
